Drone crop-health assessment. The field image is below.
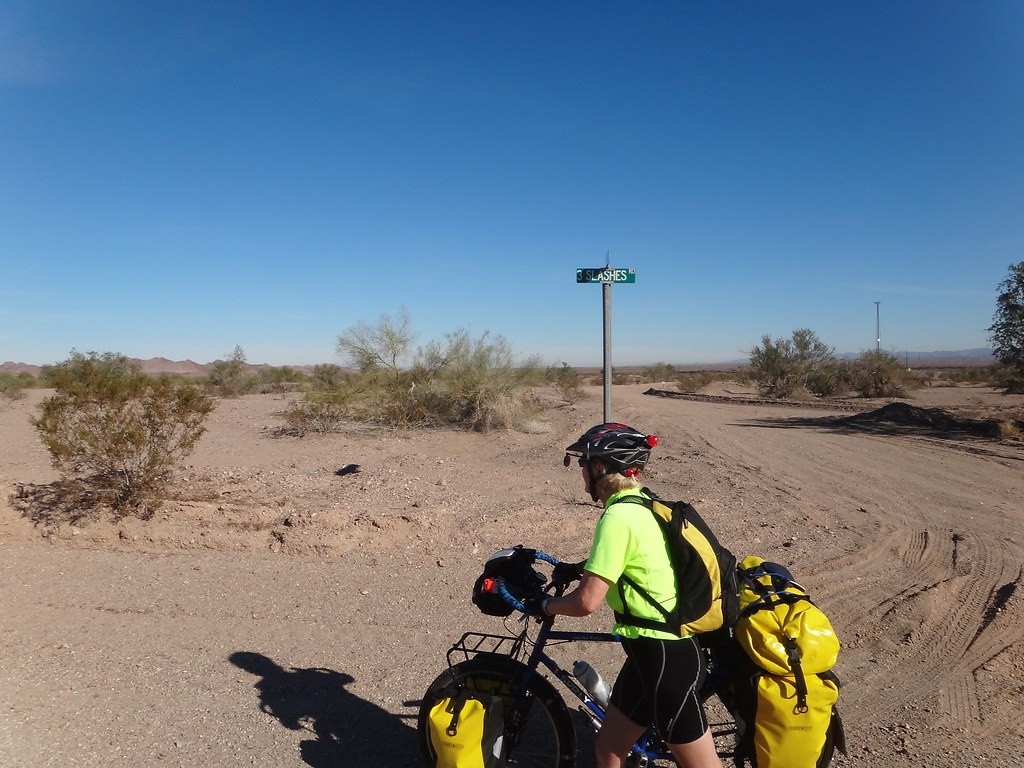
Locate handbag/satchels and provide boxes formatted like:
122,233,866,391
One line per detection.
428,688,505,768
733,554,840,768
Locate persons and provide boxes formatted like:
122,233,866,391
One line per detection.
524,422,725,768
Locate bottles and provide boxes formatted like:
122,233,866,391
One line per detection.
573,661,611,710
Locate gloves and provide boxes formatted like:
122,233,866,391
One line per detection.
551,562,582,585
522,590,555,617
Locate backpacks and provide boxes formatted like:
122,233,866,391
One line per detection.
598,488,739,637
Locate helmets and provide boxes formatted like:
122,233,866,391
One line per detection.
566,422,652,473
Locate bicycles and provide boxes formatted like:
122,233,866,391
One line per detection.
417,552,847,768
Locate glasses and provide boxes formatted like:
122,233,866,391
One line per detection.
577,458,594,467
